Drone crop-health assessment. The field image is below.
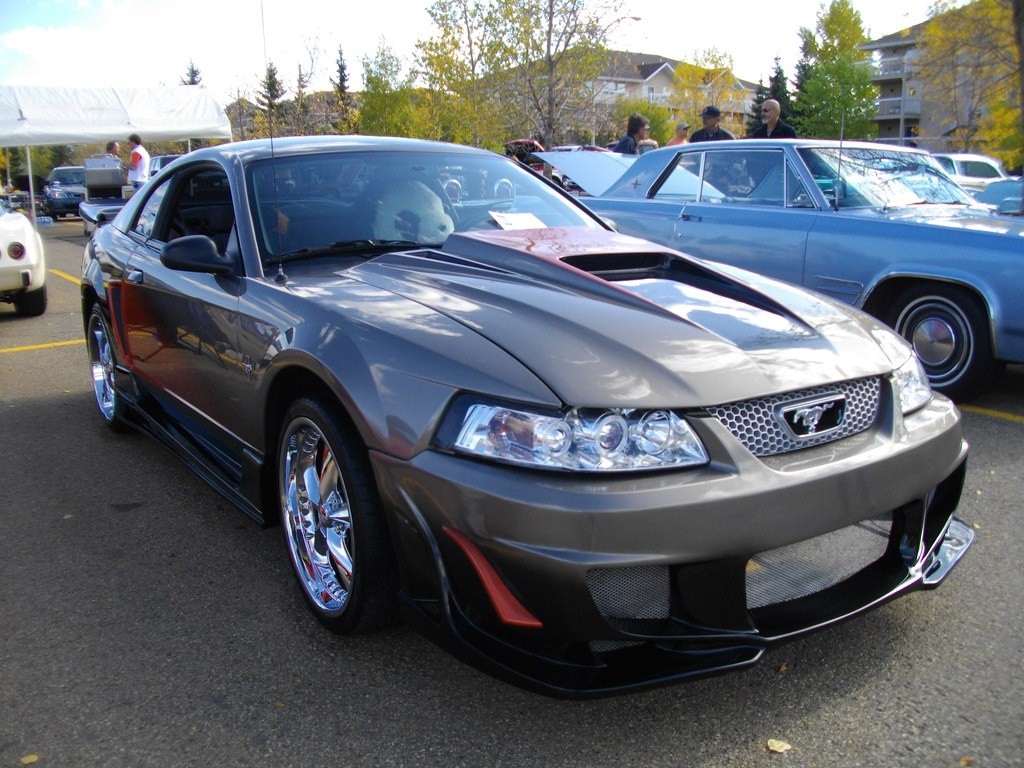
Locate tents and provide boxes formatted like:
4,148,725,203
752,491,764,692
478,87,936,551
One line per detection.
0,25,234,232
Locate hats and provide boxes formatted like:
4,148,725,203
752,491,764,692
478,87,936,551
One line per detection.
676,123,691,130
696,106,721,118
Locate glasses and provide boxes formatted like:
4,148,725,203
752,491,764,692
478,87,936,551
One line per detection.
759,108,775,112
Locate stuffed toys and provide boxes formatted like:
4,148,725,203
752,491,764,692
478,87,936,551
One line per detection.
370,177,459,244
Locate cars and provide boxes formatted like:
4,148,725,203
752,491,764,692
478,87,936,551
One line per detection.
81,133,974,701
529,137,1021,399
40,165,88,218
146,155,184,180
503,139,613,189
0,199,48,318
929,152,1022,218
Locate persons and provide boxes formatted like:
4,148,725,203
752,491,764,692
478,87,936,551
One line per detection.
687,106,737,143
106,140,122,158
615,111,651,153
665,121,690,146
752,99,798,138
125,134,150,198
